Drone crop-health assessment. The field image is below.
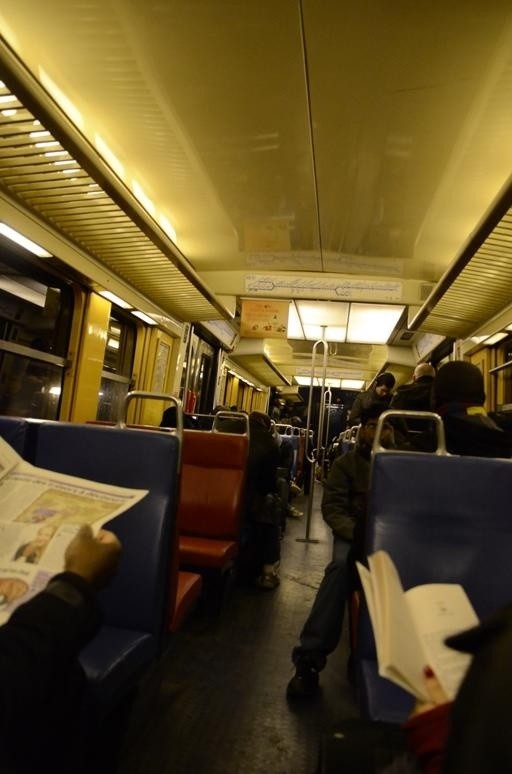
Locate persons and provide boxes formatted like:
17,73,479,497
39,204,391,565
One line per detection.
160,358,511,774
1,524,122,774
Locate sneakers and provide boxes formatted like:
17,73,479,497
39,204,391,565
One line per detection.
286,503,305,519
257,564,281,588
286,663,320,704
